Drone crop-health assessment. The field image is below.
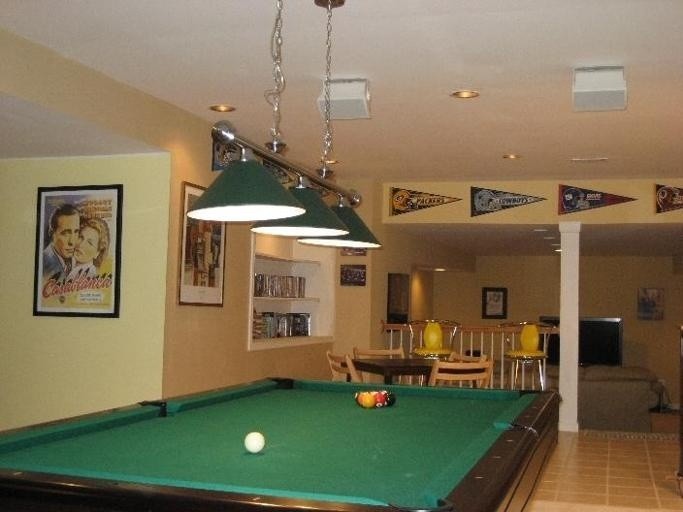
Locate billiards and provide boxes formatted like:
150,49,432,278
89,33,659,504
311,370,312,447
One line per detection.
245,432,264,452
354,391,396,407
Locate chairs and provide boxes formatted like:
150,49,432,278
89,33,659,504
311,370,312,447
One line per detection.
352,346,404,359
428,360,494,389
326,351,361,382
501,321,552,391
409,319,459,386
449,354,487,362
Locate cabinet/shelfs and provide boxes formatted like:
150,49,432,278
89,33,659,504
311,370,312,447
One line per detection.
252,253,334,344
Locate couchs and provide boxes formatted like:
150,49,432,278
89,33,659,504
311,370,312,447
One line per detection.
517,365,664,433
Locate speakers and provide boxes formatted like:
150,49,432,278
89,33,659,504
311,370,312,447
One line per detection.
317,75,373,119
571,63,627,109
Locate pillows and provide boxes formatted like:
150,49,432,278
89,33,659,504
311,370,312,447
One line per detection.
585,365,658,383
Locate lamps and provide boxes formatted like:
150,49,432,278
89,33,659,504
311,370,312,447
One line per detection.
186,0,382,249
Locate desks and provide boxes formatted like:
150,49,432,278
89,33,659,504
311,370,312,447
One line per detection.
340,358,484,388
383,322,559,388
1,377,563,512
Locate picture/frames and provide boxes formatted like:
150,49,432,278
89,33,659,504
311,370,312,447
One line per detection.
32,184,122,318
178,180,225,306
481,287,506,319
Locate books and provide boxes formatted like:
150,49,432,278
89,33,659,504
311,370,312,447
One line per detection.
251,311,310,340
253,273,306,298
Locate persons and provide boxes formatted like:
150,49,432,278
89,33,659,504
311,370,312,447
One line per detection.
65,217,108,284
42,204,80,292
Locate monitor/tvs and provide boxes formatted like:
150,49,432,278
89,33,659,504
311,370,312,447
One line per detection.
537,314,625,368
